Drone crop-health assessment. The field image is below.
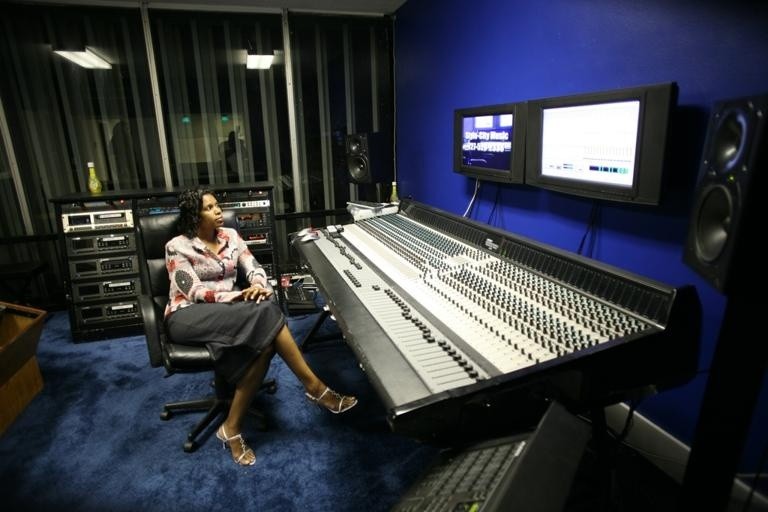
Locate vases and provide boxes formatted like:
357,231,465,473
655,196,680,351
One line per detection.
391,181,398,204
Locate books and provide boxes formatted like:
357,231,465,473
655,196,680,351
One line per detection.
344,199,386,210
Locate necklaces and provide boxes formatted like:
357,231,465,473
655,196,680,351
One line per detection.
201,234,220,253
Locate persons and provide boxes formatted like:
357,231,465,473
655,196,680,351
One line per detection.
160,186,359,468
216,129,251,175
214,158,235,174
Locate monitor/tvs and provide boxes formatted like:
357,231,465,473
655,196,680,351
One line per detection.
454,101,525,185
525,81,679,207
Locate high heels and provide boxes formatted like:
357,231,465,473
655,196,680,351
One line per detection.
302,385,359,417
215,422,257,466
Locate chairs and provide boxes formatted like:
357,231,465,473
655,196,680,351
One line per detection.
133,205,278,453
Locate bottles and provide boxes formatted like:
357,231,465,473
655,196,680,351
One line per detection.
389,182,398,203
86,161,103,193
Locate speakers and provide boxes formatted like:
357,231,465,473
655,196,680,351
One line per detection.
682,93,768,296
343,131,389,184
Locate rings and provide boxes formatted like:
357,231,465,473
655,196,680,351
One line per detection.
254,287,259,291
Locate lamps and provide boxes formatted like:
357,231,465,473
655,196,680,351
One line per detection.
51,45,113,73
246,49,276,71
